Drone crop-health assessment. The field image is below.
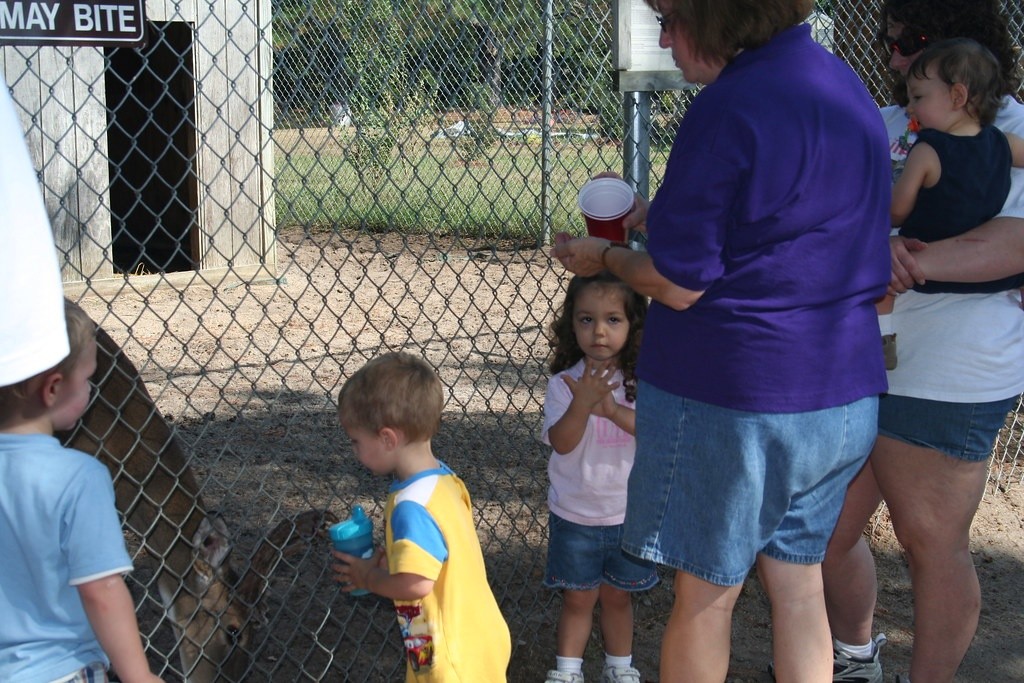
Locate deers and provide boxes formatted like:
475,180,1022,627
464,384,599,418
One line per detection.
52,295,338,683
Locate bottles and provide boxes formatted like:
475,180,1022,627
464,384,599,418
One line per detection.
328,506,375,596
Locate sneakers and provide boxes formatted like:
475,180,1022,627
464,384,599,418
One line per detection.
601,667,640,683
768,633,888,683
544,668,584,683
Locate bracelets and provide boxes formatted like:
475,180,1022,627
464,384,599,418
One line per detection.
602,240,632,274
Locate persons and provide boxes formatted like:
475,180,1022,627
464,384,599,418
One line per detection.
546,0,897,683
323,351,513,683
764,0,1023,682
0,71,73,390
0,297,164,682
537,270,660,682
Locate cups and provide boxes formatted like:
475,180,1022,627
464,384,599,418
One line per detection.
579,178,634,245
